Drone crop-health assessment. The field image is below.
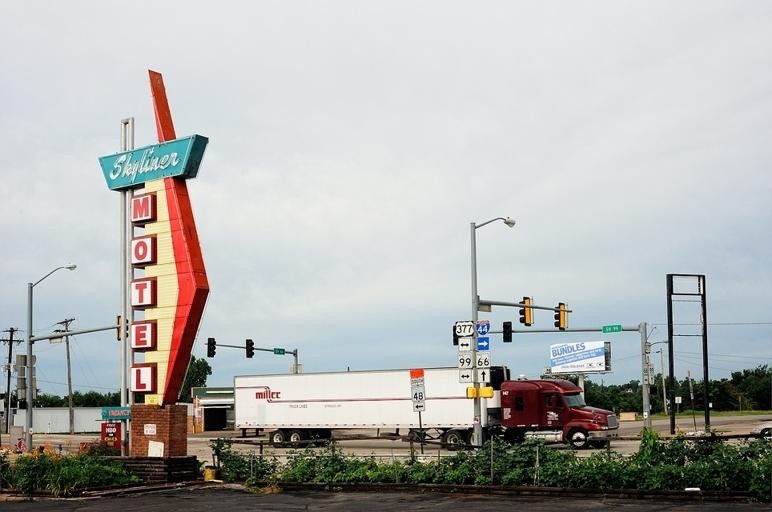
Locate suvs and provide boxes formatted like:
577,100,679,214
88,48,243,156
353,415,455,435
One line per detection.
748,422,772,446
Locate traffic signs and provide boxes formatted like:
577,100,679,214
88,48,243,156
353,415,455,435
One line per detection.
274,348,285,354
601,323,622,334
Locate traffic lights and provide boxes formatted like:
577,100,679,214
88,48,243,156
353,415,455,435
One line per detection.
245,339,255,357
466,387,476,399
502,321,512,343
117,315,129,339
555,303,565,330
207,338,217,357
519,297,532,327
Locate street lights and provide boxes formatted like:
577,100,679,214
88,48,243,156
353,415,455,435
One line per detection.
655,347,667,415
466,216,521,446
25,262,79,450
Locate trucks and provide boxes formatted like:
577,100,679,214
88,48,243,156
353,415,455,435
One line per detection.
234,368,619,452
